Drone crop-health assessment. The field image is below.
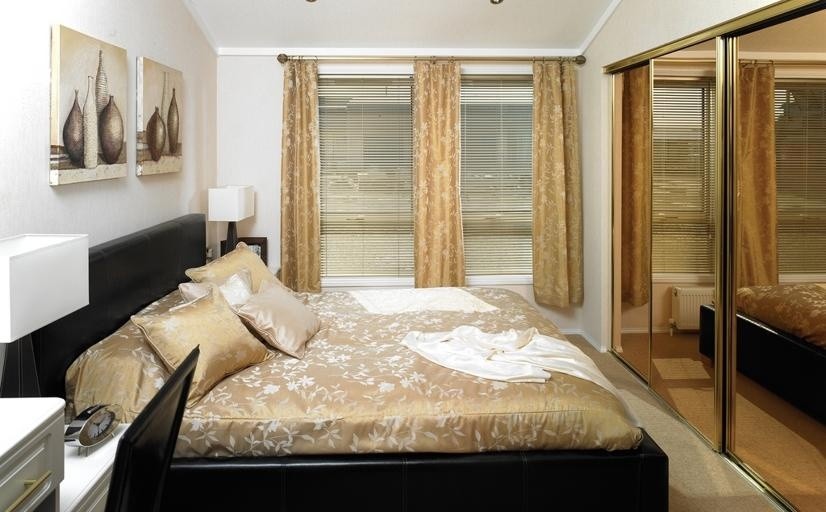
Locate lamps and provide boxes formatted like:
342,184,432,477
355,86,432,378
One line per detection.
209,185,257,255
2,235,94,400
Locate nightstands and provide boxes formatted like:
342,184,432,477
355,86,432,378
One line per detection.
1,388,132,511
268,265,281,281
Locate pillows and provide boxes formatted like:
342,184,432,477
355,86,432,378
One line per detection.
127,286,269,413
235,277,324,360
175,266,254,310
182,240,281,287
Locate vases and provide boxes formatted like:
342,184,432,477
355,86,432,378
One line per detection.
61,43,182,169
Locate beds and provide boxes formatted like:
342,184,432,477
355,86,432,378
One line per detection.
701,278,826,426
6,213,671,512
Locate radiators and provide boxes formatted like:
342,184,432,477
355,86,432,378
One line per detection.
668,284,716,333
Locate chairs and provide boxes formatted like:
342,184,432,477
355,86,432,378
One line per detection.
107,342,200,511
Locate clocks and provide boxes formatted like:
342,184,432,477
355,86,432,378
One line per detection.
67,402,124,455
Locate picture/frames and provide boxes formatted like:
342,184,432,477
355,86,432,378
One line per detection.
220,237,268,265
